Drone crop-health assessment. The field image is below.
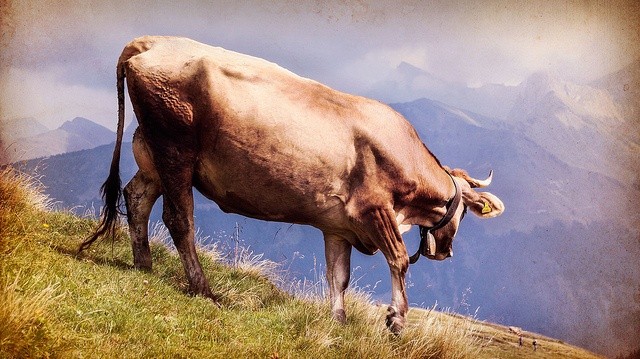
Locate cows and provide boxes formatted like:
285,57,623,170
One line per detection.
75,34,506,341
